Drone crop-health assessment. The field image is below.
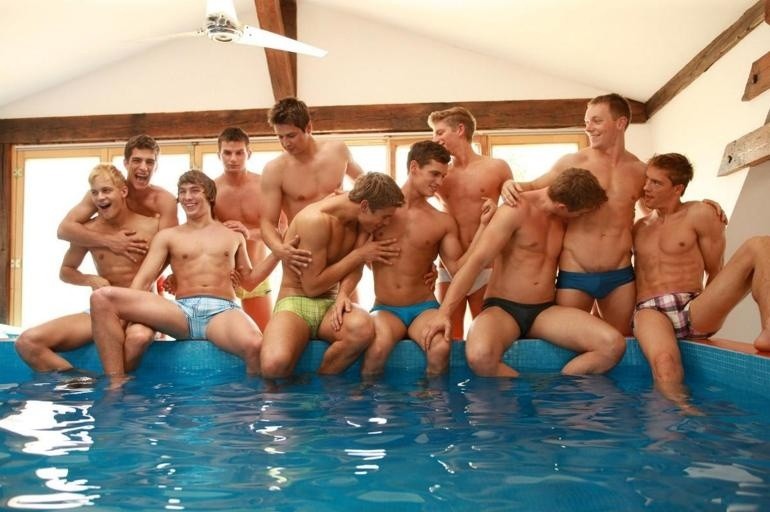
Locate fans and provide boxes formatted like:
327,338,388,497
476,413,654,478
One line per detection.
142,1,328,62
